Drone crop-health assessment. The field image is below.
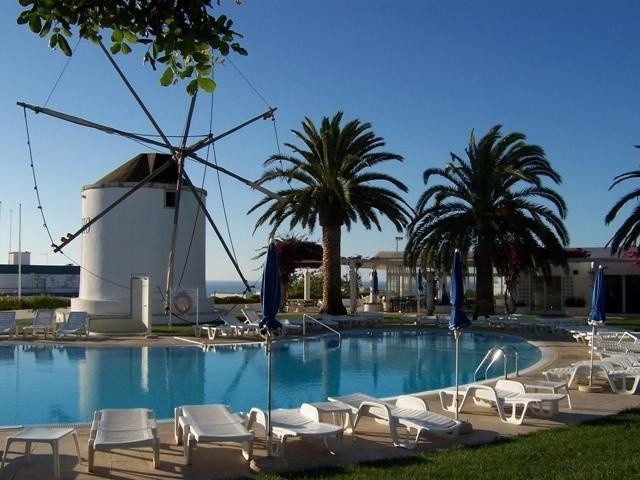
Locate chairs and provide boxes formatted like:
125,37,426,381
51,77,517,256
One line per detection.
1,428,81,478
220,315,258,336
88,407,159,472
175,404,254,466
328,393,460,451
542,309,640,394
300,402,355,441
23,310,55,340
524,379,571,411
54,313,90,341
238,408,344,459
439,379,569,425
242,309,262,335
193,325,234,340
1,311,17,340
278,309,541,336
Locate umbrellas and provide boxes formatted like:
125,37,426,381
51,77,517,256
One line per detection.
417,268,423,315
587,264,606,386
256,236,287,456
447,247,469,420
370,269,381,303
